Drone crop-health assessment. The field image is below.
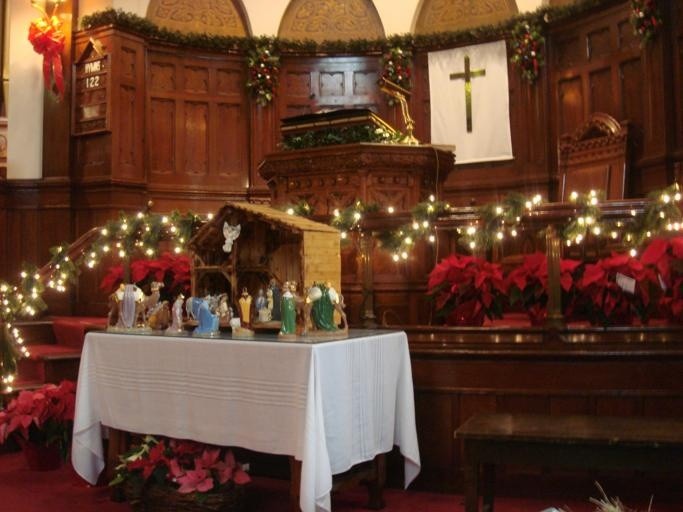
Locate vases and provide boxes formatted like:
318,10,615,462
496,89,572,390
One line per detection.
127,484,241,512
28,421,67,470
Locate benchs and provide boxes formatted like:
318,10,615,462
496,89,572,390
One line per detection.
454,413,683,512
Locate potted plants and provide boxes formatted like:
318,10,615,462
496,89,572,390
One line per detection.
0,379,76,441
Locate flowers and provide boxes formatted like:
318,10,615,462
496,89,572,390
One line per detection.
424,235,683,330
99,250,191,321
244,43,281,109
379,47,415,108
112,435,252,501
508,22,547,88
626,0,664,50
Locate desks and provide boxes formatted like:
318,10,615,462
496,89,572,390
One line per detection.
72,330,421,512
